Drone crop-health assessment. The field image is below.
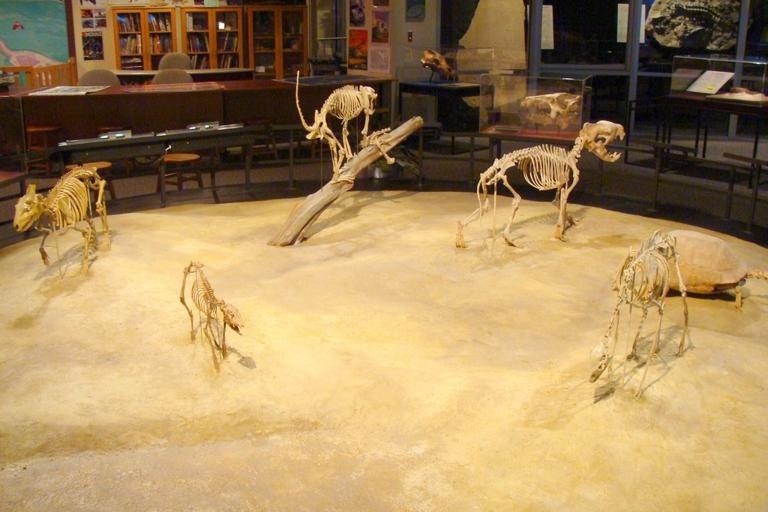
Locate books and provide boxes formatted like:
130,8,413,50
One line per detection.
116,12,241,71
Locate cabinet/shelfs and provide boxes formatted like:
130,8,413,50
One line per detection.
111,5,311,78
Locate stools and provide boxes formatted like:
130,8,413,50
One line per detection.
25,125,65,177
64,160,117,202
98,125,136,167
156,153,203,192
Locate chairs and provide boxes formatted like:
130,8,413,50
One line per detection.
77,69,121,87
151,68,195,83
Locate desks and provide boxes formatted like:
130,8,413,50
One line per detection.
398,79,495,155
56,124,256,204
